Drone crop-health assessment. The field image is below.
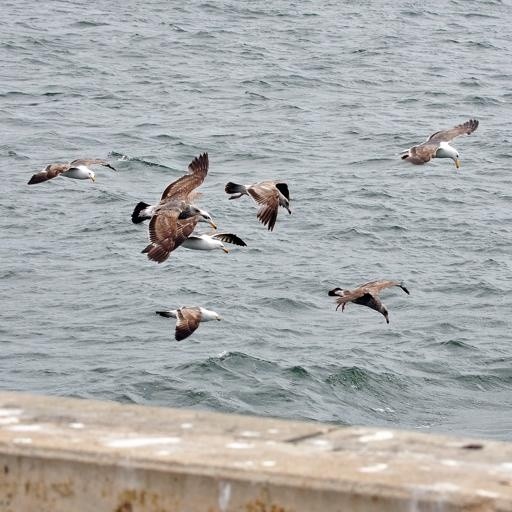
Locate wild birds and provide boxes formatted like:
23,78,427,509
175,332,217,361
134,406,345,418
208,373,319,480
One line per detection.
225,179,292,231
27,158,118,186
155,305,223,342
328,280,410,324
400,118,478,170
131,151,217,264
180,231,247,255
